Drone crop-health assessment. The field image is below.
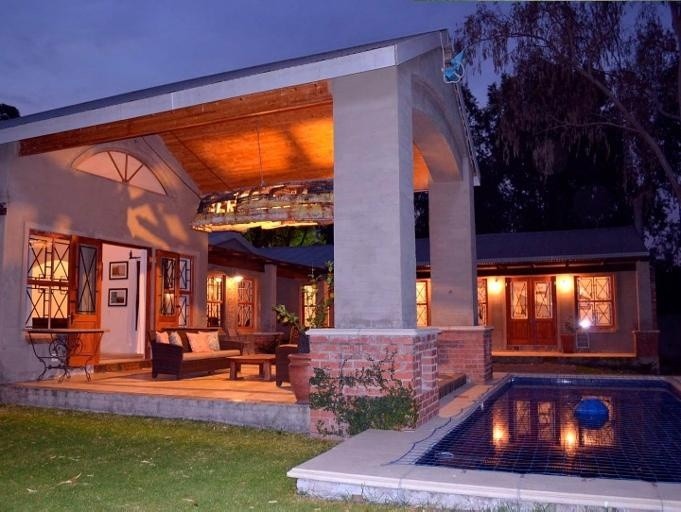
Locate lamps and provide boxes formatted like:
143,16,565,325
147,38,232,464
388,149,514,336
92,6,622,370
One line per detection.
189,115,421,238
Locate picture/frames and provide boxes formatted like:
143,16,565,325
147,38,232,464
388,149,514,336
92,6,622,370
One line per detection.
108,287,128,307
108,260,129,280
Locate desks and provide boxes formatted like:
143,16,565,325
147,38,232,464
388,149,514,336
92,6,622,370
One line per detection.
21,326,110,383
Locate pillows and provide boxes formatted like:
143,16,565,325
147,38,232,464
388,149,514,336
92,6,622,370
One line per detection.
198,329,221,351
168,331,185,347
156,330,170,345
184,331,211,353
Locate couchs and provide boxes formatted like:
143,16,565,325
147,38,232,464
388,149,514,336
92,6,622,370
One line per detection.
277,326,333,390
147,328,245,377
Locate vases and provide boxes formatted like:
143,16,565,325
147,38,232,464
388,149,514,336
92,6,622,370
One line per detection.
287,353,314,405
